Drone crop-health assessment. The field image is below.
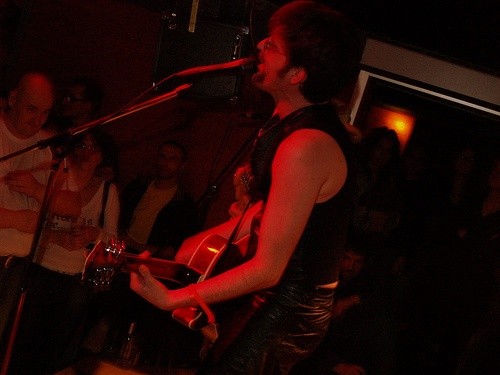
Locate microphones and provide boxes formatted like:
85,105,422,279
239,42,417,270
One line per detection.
174,56,259,79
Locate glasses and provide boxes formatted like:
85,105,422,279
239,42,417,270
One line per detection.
76,143,101,152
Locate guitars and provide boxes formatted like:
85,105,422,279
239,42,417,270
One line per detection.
81,234,249,330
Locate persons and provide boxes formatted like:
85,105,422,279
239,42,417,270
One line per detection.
41,127,121,249
359,127,400,171
129,0,364,374
119,142,194,255
62,80,123,193
1,73,82,303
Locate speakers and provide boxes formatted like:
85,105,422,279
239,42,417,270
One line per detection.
150,14,245,100
